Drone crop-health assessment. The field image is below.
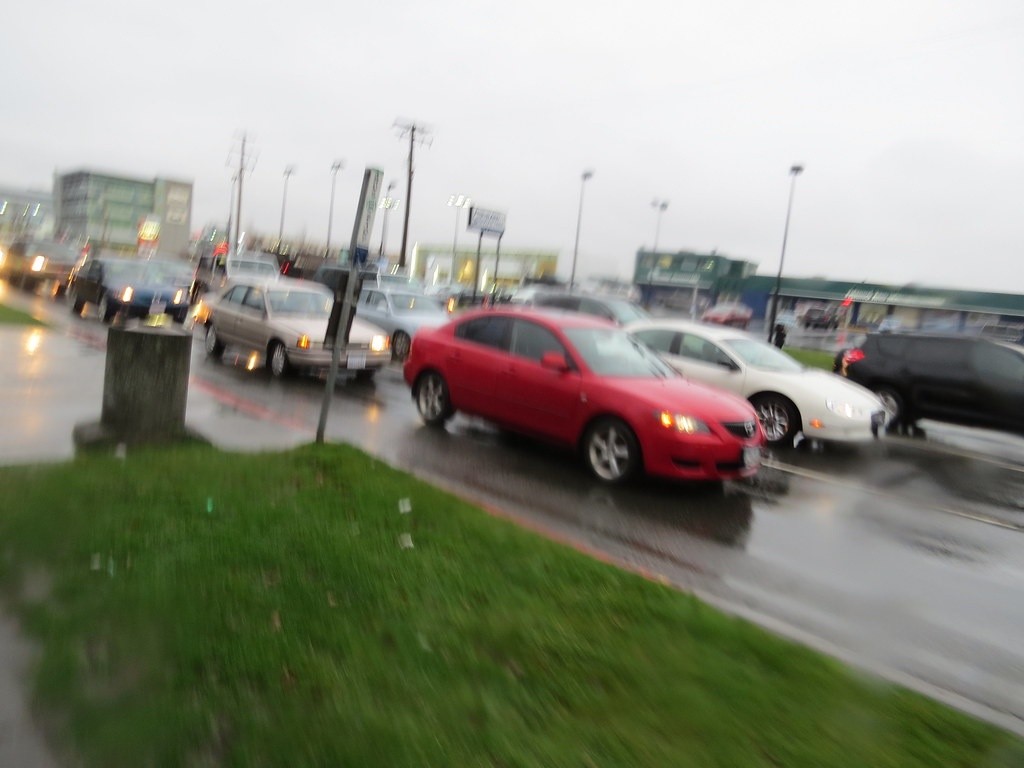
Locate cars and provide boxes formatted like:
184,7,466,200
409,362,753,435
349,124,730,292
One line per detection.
2,230,643,329
71,256,193,326
354,278,453,363
610,316,896,455
196,271,393,384
700,302,755,329
836,327,1024,443
403,305,770,490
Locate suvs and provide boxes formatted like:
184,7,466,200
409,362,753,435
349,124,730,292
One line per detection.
803,307,839,331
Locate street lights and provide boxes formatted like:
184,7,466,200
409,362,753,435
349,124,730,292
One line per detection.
768,162,805,343
643,200,669,312
389,117,433,269
569,167,594,292
278,165,299,254
326,156,349,263
447,193,472,288
